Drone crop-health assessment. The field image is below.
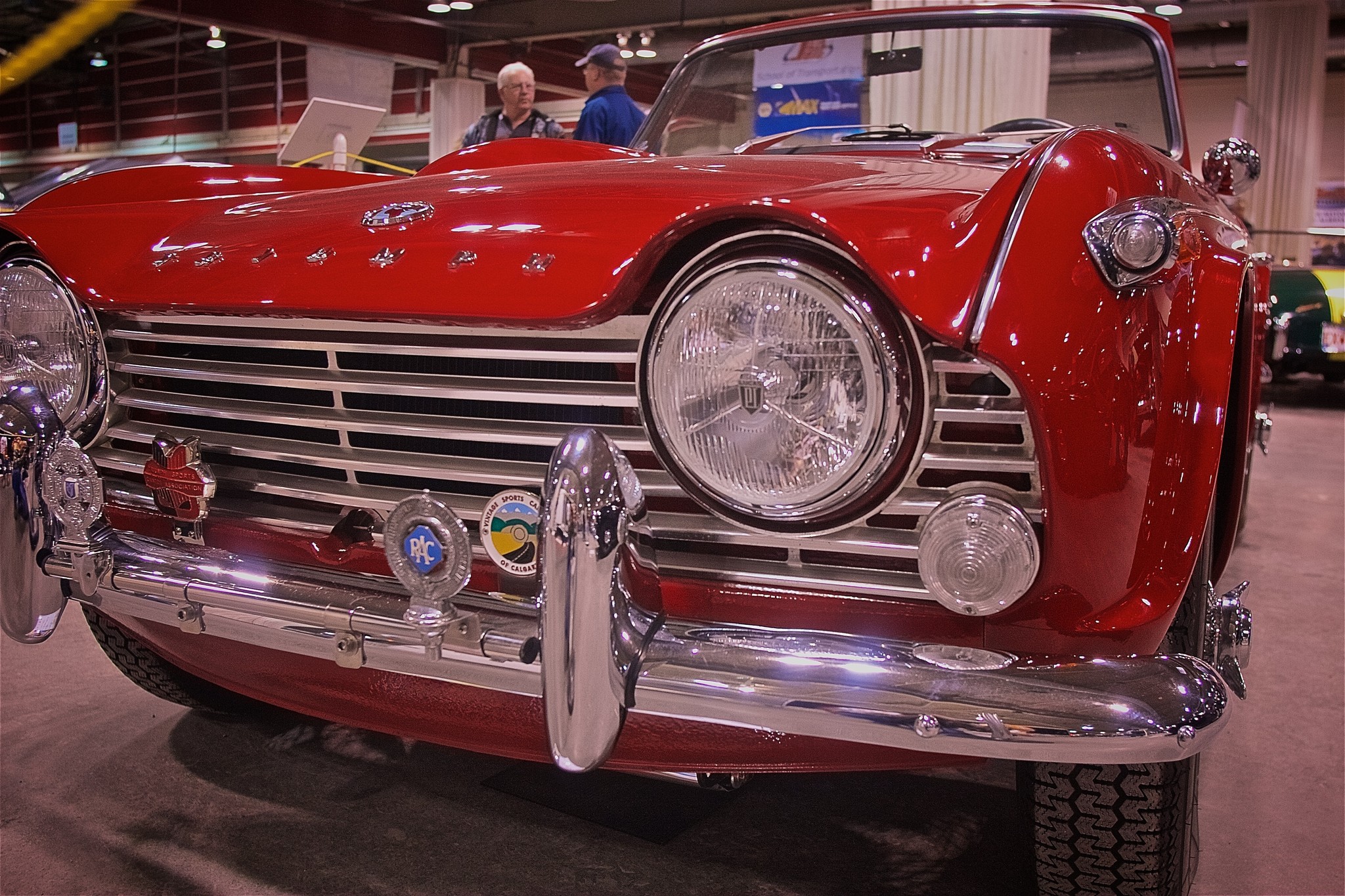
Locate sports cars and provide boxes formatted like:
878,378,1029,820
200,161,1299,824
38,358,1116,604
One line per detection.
1261,256,1344,386
1,0,1276,895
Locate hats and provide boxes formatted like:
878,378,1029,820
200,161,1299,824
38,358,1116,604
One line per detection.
575,44,627,73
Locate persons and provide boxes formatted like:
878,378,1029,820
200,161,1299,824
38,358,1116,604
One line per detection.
575,42,663,154
462,61,567,148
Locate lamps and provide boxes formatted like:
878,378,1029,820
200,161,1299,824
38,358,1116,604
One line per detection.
206,26,226,48
617,34,633,58
636,29,656,58
